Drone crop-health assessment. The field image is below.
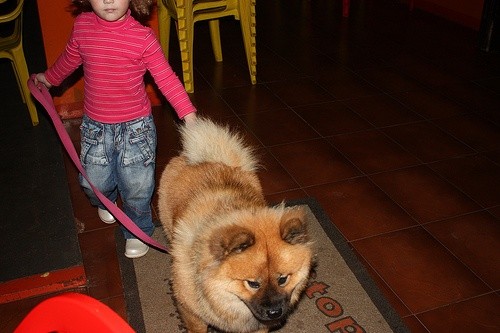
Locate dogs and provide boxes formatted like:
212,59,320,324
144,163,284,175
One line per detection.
158,116,316,333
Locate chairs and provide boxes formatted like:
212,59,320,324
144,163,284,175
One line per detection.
158,0,257,94
0,0,40,127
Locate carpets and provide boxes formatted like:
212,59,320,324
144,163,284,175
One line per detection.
0,24,90,304
114,196,413,333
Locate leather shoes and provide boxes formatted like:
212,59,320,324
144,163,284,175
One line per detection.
97,201,118,224
124,238,150,259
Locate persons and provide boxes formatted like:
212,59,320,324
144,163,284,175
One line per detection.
34,0,198,258
